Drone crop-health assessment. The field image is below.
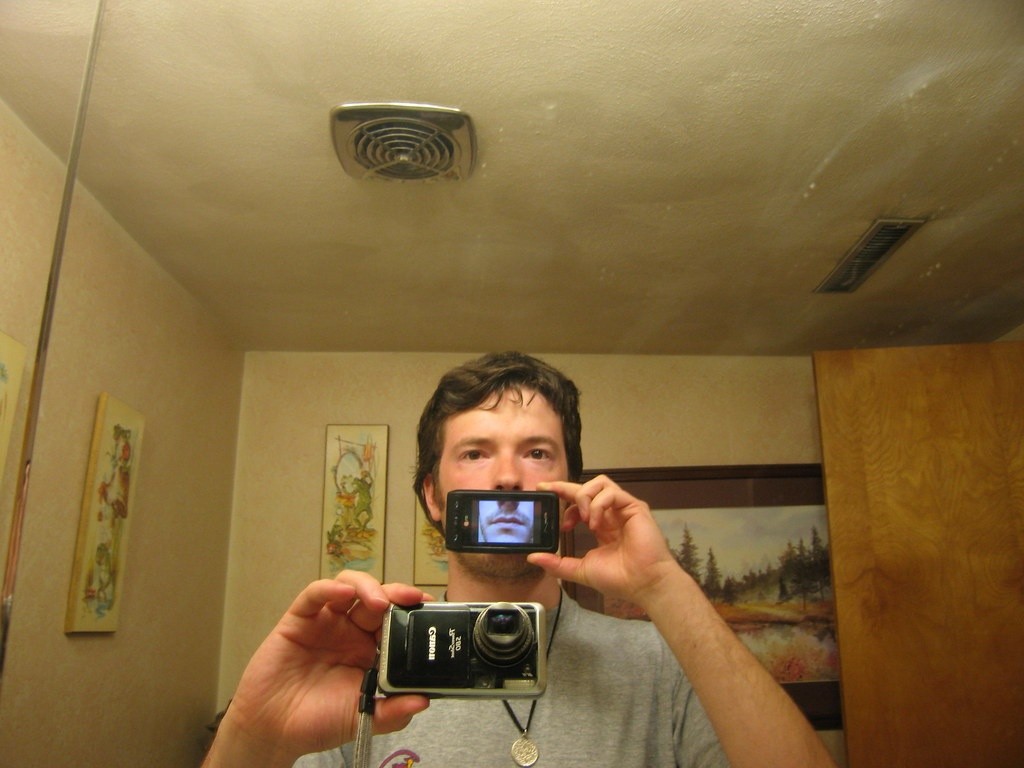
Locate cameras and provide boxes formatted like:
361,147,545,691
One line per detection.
378,601,547,700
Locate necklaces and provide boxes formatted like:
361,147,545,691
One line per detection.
444,589,564,767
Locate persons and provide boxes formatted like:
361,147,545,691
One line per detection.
201,352,839,768
479,501,533,542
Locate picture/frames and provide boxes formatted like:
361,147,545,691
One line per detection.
559,462,844,733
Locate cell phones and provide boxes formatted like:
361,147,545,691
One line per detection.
446,489,559,554
